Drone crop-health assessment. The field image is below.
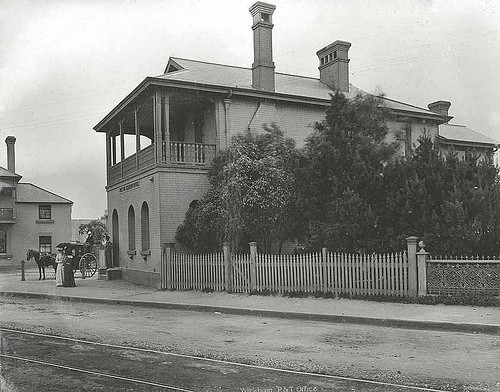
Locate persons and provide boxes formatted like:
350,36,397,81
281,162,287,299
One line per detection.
104,236,114,269
62,247,78,287
55,248,66,287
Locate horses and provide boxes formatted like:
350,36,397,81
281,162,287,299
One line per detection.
26,249,57,280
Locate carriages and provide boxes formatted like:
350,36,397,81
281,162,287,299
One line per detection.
55,241,98,278
